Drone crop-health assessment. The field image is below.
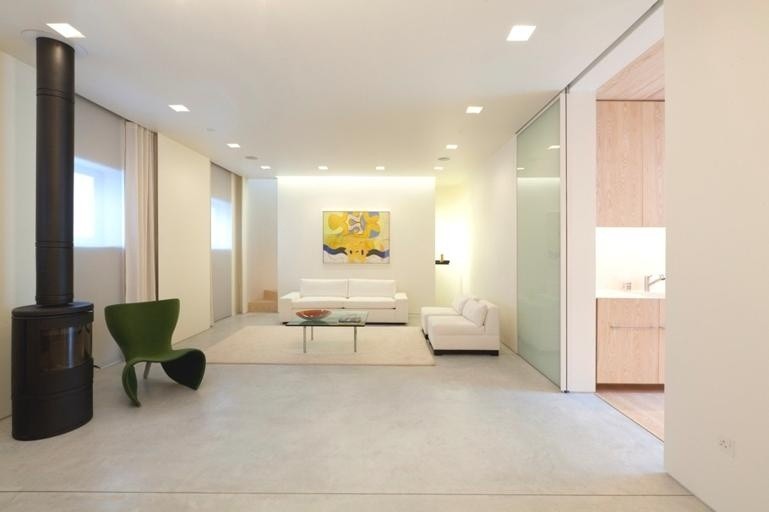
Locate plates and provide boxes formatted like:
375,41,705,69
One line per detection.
297,309,331,321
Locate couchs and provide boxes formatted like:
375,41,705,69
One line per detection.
279,278,408,324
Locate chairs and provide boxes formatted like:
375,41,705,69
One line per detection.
420,293,499,356
105,300,206,407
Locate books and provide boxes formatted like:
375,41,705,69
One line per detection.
339,319,361,323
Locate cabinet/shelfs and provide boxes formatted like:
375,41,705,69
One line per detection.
598,97,666,226
598,298,664,386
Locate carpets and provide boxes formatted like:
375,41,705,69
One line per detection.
203,325,435,367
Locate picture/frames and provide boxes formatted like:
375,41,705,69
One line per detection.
322,211,390,264
